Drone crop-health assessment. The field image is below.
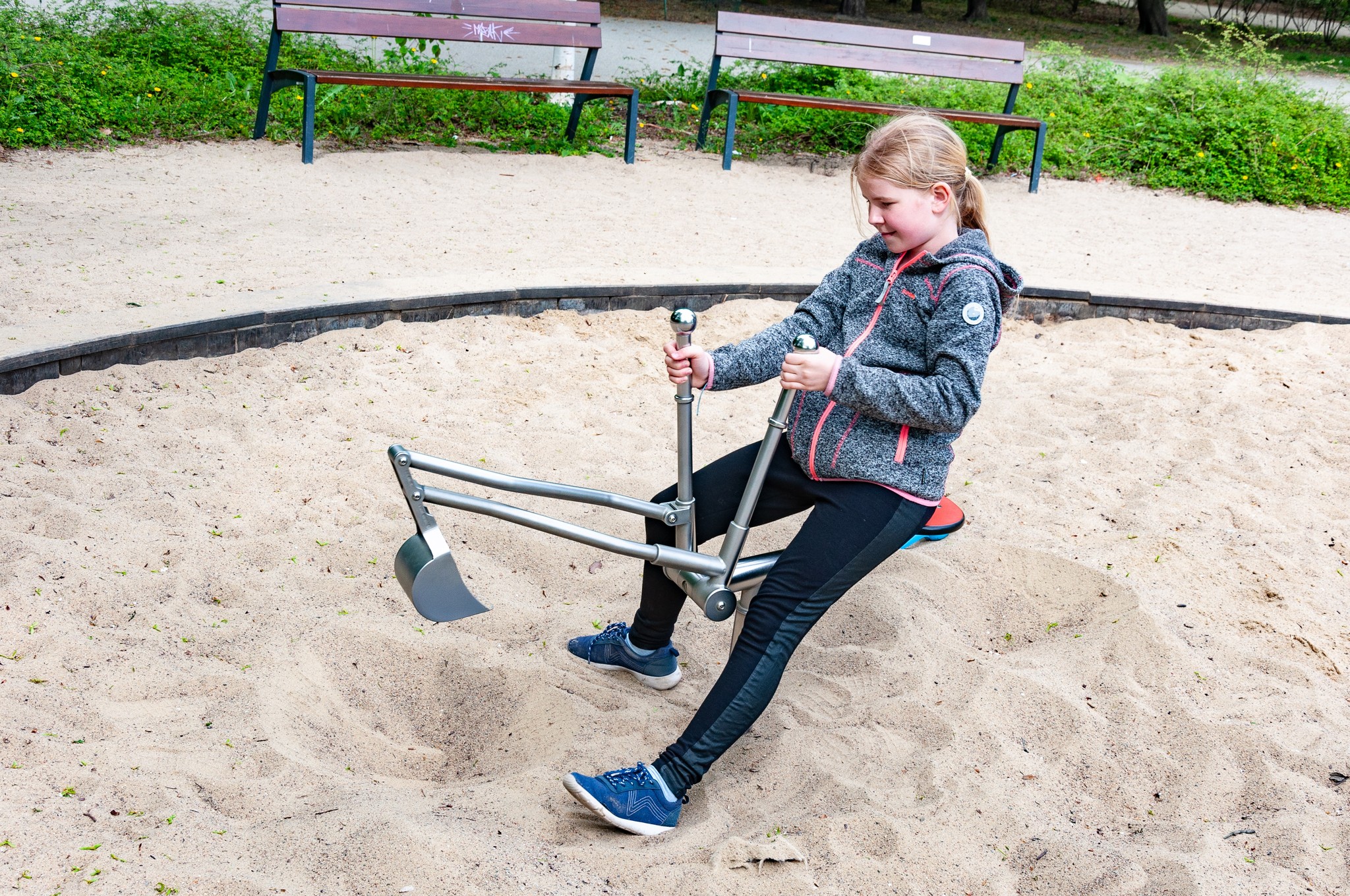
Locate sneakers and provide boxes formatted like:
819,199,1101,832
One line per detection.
562,761,691,835
567,621,683,690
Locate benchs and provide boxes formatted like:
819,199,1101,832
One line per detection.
696,10,1046,196
252,0,640,167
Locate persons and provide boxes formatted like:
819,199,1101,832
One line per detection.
563,112,1023,836
